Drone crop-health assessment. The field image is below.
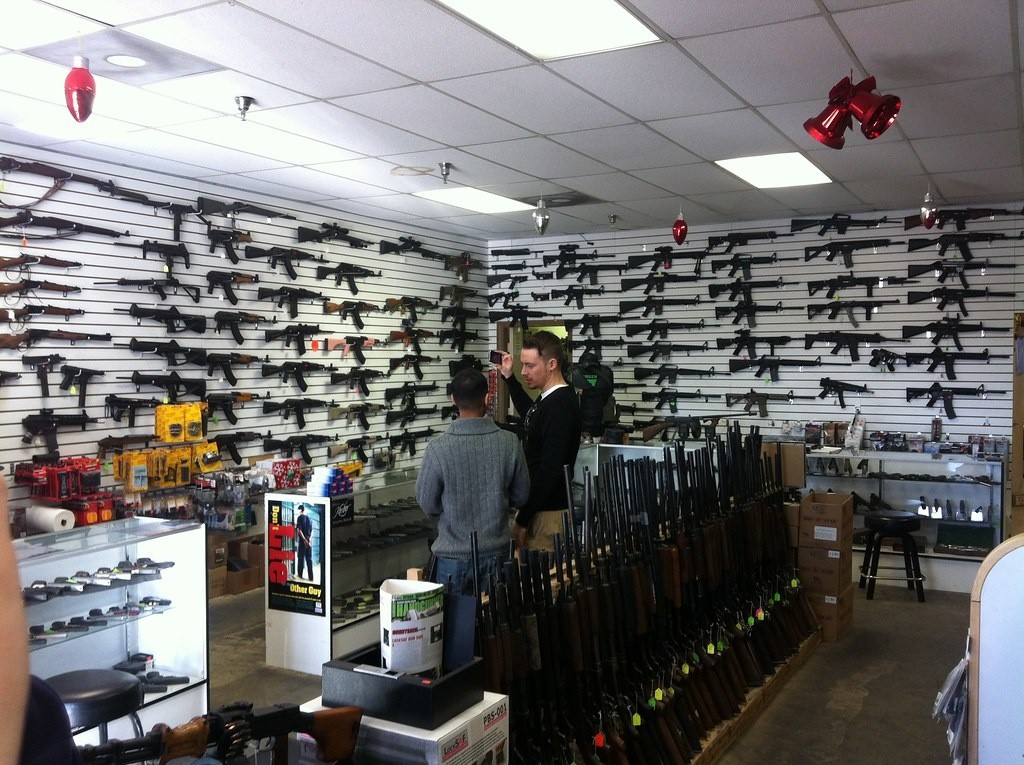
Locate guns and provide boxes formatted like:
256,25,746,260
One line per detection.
331,497,438,624
23,555,190,694
0,156,486,464
75,701,363,765
812,456,1003,558
484,207,1024,440
466,420,821,765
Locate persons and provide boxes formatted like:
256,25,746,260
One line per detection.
415,367,530,598
295,505,315,581
487,330,581,553
0,472,83,765
567,353,614,437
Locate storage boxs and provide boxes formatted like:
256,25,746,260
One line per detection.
784,502,800,547
797,547,852,595
798,493,853,550
806,589,853,643
207,541,264,599
299,690,509,765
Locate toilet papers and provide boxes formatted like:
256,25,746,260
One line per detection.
25,505,76,532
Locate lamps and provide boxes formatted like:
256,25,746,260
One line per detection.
803,69,902,149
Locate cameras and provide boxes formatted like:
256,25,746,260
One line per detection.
489,350,502,365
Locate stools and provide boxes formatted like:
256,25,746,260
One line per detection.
858,510,927,603
45,669,144,745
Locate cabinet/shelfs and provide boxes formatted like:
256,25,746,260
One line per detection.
799,449,1004,593
12,517,209,747
263,469,439,676
